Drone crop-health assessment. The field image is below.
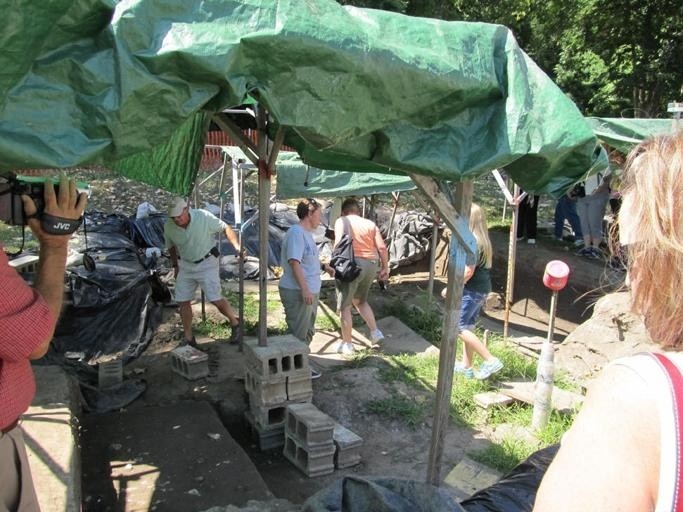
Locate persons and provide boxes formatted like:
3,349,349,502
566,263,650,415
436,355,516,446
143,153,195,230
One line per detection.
0,176,88,512
606,148,628,269
441,204,504,379
334,199,389,355
568,171,612,259
278,199,336,379
533,131,683,512
164,197,247,348
555,195,584,246
516,189,539,244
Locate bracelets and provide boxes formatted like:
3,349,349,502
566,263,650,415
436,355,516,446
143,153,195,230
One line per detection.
172,264,178,267
322,264,325,270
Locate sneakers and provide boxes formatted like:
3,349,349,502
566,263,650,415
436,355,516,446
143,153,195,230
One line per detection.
369,328,384,346
174,335,195,349
573,247,591,256
334,341,355,355
572,238,584,247
583,248,601,259
230,317,241,344
516,235,525,241
451,363,473,378
527,238,535,244
309,368,323,380
474,356,504,380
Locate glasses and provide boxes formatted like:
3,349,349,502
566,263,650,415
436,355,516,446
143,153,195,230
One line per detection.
615,238,662,271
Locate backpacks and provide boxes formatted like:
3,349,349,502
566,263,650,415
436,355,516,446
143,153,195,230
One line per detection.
328,216,361,282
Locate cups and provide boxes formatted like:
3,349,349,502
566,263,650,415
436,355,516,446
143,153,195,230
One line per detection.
542,259,570,291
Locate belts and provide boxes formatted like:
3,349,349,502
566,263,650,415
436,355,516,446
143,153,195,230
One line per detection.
193,247,213,265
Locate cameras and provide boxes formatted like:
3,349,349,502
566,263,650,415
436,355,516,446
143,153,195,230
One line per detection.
0,170,96,235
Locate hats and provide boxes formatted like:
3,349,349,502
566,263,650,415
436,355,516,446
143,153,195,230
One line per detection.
166,197,187,218
